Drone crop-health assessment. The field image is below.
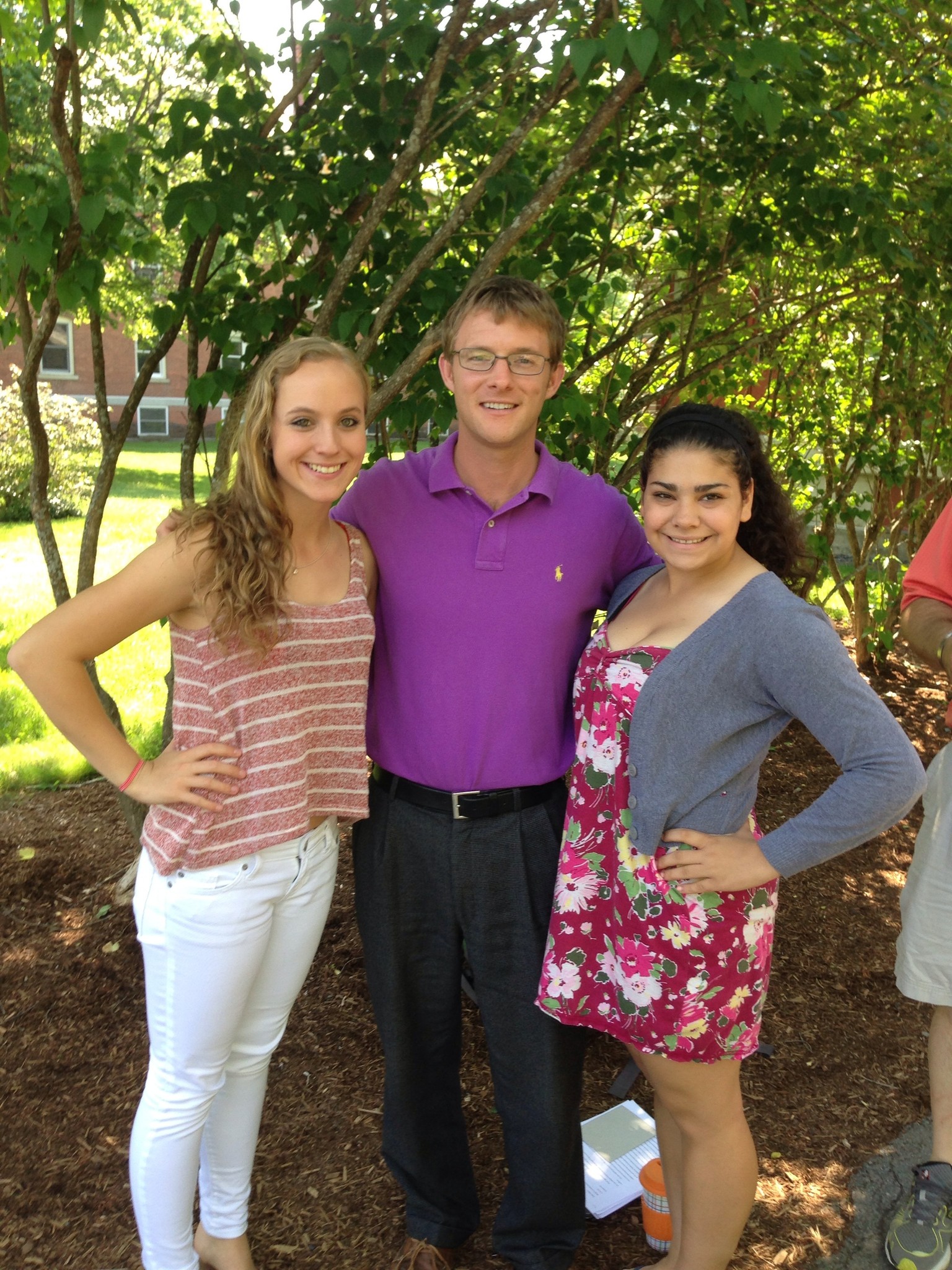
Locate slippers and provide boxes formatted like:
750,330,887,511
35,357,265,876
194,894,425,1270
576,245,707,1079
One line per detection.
631,1264,650,1270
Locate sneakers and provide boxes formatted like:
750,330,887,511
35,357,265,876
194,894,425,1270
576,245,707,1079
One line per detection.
886,1162,952,1270
472,1252,519,1270
390,1237,459,1270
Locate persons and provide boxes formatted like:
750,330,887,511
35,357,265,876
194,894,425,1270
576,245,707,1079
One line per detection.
13,336,378,1270
872,490,952,1270
537,404,929,1270
157,275,656,1270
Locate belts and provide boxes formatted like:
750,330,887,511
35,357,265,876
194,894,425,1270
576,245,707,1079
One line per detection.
371,764,566,820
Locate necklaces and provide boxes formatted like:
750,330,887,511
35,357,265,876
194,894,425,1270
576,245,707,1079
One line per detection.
281,526,333,575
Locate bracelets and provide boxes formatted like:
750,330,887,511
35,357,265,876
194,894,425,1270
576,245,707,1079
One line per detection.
937,632,952,670
117,759,144,792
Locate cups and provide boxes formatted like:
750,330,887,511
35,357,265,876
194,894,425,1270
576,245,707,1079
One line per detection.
639,1158,672,1252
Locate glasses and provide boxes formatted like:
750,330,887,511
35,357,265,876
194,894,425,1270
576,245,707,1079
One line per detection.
449,346,554,376
